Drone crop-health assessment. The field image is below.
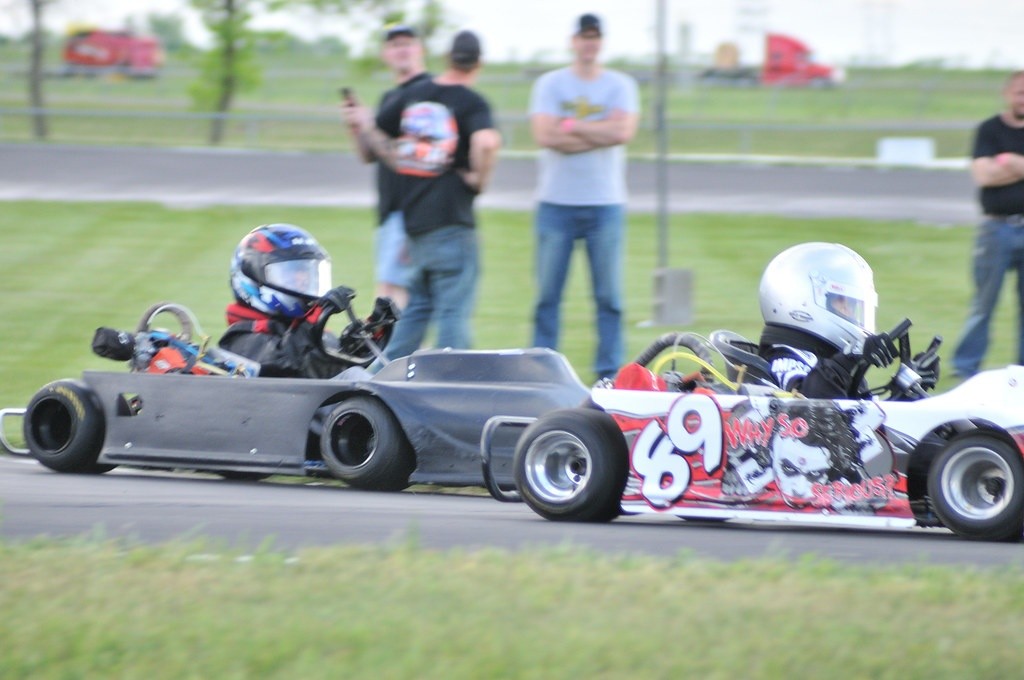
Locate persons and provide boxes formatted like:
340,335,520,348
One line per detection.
950,72,1024,376
758,242,940,399
341,27,500,374
218,224,396,379
531,13,639,381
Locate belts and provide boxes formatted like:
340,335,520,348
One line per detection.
987,212,1024,227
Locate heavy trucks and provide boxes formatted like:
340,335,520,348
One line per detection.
703,30,844,94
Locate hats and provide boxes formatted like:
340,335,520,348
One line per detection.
569,15,603,41
451,30,479,63
381,21,416,40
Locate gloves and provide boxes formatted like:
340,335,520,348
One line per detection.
368,297,403,325
862,332,899,368
913,352,939,390
319,286,356,313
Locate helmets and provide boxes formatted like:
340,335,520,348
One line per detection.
229,224,332,317
759,242,879,355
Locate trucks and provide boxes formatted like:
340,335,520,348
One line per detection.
67,33,163,81
